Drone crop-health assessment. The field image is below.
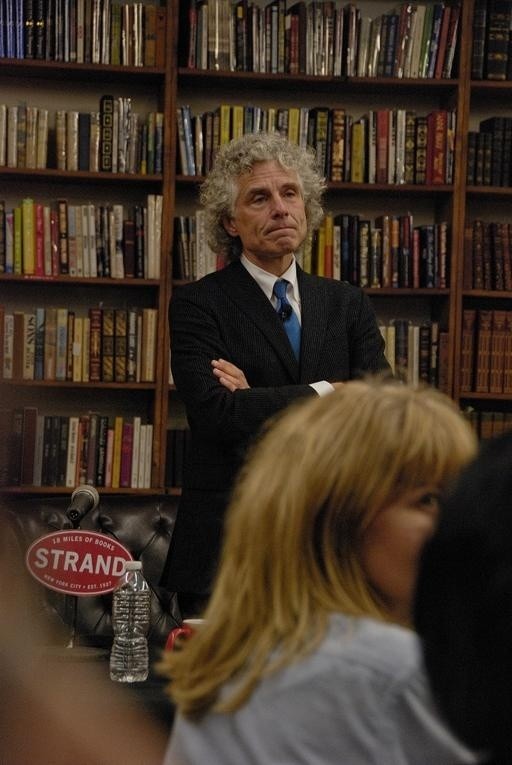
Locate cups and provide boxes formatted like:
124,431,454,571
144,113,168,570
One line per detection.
165,618,208,653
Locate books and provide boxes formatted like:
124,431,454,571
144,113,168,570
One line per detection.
3,5,511,496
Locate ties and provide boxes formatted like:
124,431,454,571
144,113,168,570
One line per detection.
273,278,302,363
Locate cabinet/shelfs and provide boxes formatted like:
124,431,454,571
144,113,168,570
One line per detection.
0,0,512,495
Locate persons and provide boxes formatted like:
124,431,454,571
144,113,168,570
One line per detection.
405,434,510,765
144,368,483,764
157,128,394,621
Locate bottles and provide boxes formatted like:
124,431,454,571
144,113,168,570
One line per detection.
108,558,150,684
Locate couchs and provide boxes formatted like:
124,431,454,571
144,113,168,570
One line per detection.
0,493,187,736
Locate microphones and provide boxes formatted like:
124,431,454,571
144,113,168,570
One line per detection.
65,484,100,522
